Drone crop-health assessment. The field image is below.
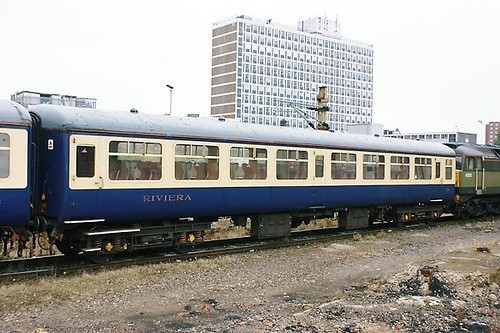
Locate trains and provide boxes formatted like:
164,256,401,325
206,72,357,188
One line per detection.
0,100,500,260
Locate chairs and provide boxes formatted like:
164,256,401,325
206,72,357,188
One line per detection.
107,163,413,184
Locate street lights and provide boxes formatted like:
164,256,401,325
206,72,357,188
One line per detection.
166,84,174,115
